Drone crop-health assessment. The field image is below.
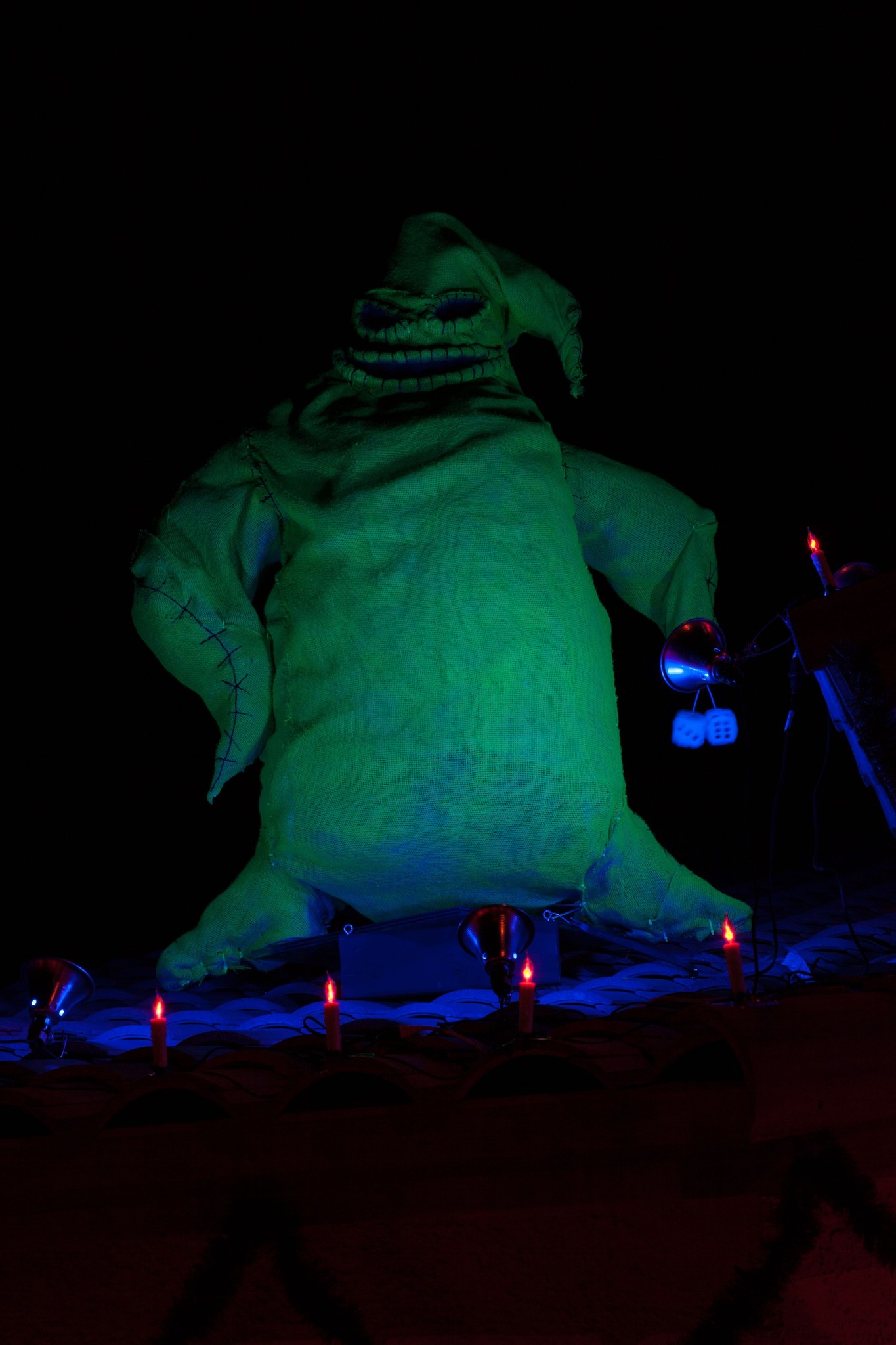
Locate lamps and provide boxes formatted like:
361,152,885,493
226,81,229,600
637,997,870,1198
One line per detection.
457,903,534,1016
22,959,97,1061
659,617,743,695
807,530,840,590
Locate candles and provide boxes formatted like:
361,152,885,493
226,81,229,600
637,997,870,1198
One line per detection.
149,994,168,1069
323,972,343,1053
721,912,748,998
519,956,534,1033
